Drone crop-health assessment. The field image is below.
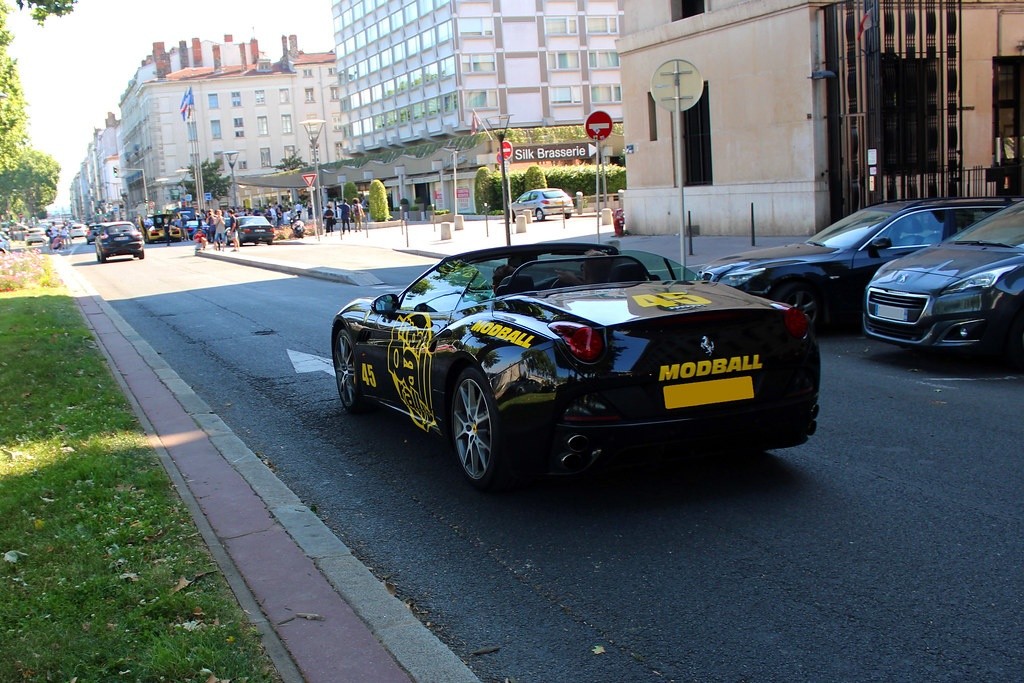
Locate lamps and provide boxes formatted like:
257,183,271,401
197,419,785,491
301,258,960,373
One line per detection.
811,71,837,80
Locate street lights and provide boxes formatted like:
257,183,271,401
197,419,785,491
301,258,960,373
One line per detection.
222,150,240,210
483,113,513,246
298,118,327,235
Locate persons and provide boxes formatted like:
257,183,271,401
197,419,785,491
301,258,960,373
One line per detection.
207,212,216,242
240,190,294,227
307,201,312,219
493,264,520,295
296,202,302,219
214,210,226,252
180,215,190,241
143,216,153,229
52,236,63,251
337,198,351,233
200,209,206,222
324,205,334,236
194,229,205,251
50,222,57,249
353,198,365,232
164,218,171,246
556,250,613,285
62,224,68,249
227,209,239,252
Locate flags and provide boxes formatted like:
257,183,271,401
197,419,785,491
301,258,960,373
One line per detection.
471,114,480,136
179,86,194,122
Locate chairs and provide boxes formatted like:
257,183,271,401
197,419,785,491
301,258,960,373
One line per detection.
497,276,535,295
899,217,943,245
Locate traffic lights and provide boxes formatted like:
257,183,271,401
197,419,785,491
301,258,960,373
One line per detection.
113,166,118,176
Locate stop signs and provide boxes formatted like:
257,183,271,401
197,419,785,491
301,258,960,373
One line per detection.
585,110,613,141
502,141,512,160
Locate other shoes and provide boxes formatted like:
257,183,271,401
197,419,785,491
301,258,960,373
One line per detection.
232,249,239,252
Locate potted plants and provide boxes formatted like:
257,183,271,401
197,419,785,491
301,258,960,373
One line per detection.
426,205,434,219
362,207,371,221
414,197,427,211
408,206,422,220
399,198,408,220
369,179,388,222
430,210,453,223
388,207,399,219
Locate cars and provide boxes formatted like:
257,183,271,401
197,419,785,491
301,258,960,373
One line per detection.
0,211,232,253
93,221,146,263
860,197,1024,374
507,187,574,222
225,215,275,246
694,197,1024,337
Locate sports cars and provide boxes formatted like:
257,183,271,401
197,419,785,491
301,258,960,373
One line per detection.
331,242,820,494
